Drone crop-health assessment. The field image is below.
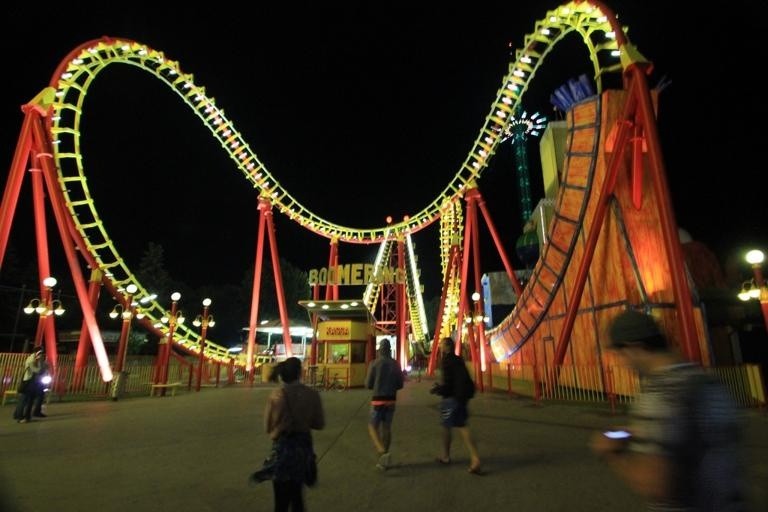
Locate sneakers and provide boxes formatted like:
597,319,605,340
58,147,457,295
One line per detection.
12,412,47,424
375,452,391,473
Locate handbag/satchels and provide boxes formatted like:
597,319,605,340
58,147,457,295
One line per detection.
303,453,318,489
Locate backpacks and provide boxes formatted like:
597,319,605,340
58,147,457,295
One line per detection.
646,372,756,511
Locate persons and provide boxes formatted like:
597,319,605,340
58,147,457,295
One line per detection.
31,360,51,418
584,308,747,512
262,357,325,511
12,347,44,423
363,339,405,472
429,336,482,474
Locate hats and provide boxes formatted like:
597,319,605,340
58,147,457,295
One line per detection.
604,309,659,349
379,339,391,350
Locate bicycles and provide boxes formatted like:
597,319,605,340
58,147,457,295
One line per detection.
315,374,345,392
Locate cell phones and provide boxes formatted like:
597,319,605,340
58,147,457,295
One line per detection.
602,429,631,441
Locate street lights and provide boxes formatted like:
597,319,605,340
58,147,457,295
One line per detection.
160,292,185,399
738,250,767,324
192,298,215,391
110,283,144,399
465,290,491,393
25,277,64,352
